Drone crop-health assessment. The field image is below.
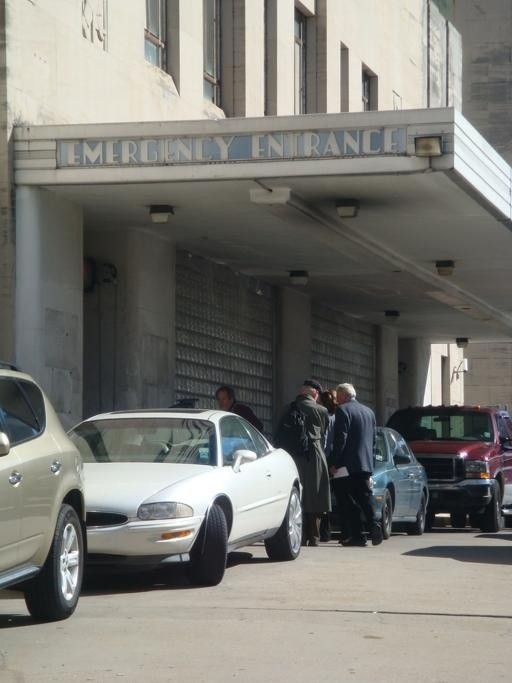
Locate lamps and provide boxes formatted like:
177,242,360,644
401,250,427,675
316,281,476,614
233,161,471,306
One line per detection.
287,269,310,288
455,337,468,348
413,134,446,169
335,198,360,218
435,259,454,277
149,203,176,224
383,308,401,321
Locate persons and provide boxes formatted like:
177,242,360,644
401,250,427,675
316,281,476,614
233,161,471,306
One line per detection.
215,386,264,432
274,379,336,548
331,383,383,547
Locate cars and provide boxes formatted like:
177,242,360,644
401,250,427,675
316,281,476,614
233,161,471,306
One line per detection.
0,361,304,620
370,404,512,539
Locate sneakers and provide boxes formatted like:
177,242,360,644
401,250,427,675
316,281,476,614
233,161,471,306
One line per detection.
371,523,384,546
302,534,332,547
341,537,370,547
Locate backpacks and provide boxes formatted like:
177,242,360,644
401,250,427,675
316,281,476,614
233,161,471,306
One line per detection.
276,402,309,454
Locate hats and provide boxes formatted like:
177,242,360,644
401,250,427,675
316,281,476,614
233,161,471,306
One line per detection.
303,379,322,394
